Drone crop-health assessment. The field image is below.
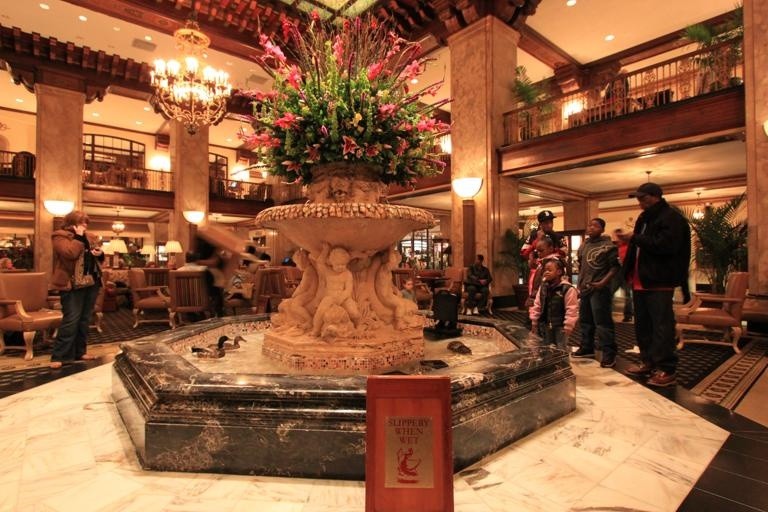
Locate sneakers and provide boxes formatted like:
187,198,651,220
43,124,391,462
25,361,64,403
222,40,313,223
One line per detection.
571,348,595,358
600,357,616,368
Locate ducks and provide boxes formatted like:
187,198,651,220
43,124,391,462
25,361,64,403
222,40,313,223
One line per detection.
192,335,233,359
208,335,247,351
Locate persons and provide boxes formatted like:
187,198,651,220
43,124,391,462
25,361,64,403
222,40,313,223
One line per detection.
0,257,14,270
48,209,104,370
518,182,693,387
307,240,360,338
374,240,418,319
290,248,318,332
399,277,418,305
464,253,494,311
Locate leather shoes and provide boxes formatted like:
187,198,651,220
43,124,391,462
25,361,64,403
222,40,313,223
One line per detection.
627,364,652,374
646,370,677,386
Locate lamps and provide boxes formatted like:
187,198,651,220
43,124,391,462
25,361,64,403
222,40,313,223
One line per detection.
112,211,125,235
44,200,74,218
149,0,232,135
183,211,205,225
452,177,482,198
101,239,184,269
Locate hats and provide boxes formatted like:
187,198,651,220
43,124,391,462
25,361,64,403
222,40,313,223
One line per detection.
537,211,556,221
628,182,662,198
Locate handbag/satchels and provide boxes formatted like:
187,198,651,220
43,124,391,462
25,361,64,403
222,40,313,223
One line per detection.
73,275,95,289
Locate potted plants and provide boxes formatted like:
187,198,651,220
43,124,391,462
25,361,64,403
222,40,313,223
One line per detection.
677,0,744,97
669,190,750,309
493,227,539,310
511,65,555,140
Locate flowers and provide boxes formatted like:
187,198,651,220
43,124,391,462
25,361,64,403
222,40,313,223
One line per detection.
235,9,454,190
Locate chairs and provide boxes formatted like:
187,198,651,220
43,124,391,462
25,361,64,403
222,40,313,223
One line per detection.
673,272,749,354
128,267,303,330
391,267,493,315
218,181,265,201
0,269,106,361
